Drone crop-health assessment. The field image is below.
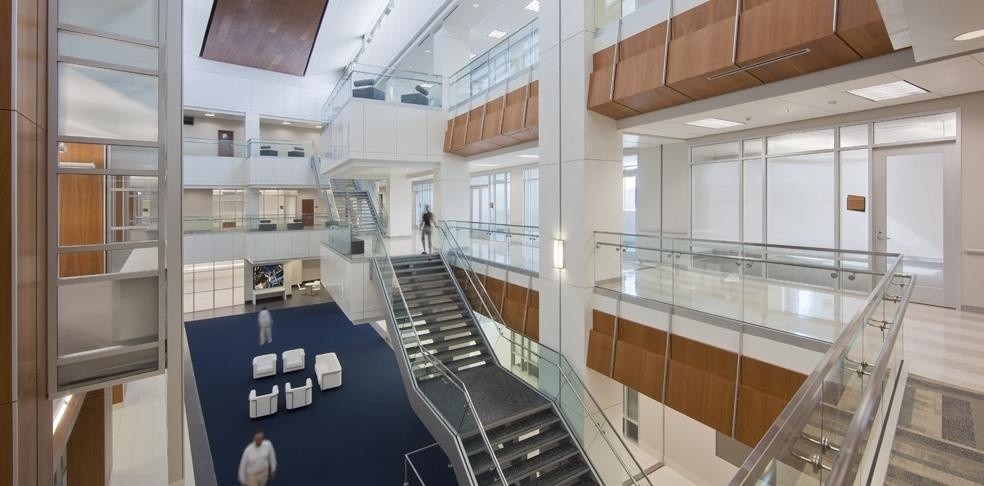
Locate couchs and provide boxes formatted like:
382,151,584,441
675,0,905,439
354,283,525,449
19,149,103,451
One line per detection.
315,352,342,391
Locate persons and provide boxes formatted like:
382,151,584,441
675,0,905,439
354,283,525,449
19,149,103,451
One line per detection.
236,428,277,486
417,202,440,259
256,304,273,346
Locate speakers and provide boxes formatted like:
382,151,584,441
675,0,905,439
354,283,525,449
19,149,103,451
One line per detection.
184,117,193,124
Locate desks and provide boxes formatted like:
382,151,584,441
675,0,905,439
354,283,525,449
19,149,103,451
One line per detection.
252,287,286,305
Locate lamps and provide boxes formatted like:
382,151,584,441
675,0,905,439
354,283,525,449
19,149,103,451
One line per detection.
552,239,565,268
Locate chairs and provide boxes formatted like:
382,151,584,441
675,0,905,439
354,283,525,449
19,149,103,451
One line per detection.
297,281,320,295
285,378,313,410
401,86,428,105
288,147,304,157
287,219,304,229
252,353,277,379
282,348,305,373
249,385,279,418
353,79,385,100
259,220,277,231
260,146,277,156
